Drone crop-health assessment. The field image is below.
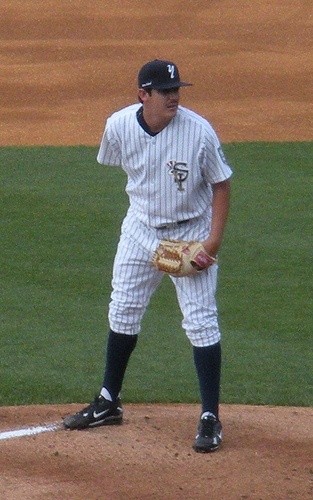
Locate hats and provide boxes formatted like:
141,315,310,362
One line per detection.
137,59,192,90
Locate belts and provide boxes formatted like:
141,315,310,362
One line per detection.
178,219,188,226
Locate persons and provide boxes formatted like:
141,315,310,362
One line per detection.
64,59,233,452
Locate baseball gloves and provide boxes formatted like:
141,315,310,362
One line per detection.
152,238,216,277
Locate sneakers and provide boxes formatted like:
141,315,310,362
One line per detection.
194,411,223,452
64,388,124,429
